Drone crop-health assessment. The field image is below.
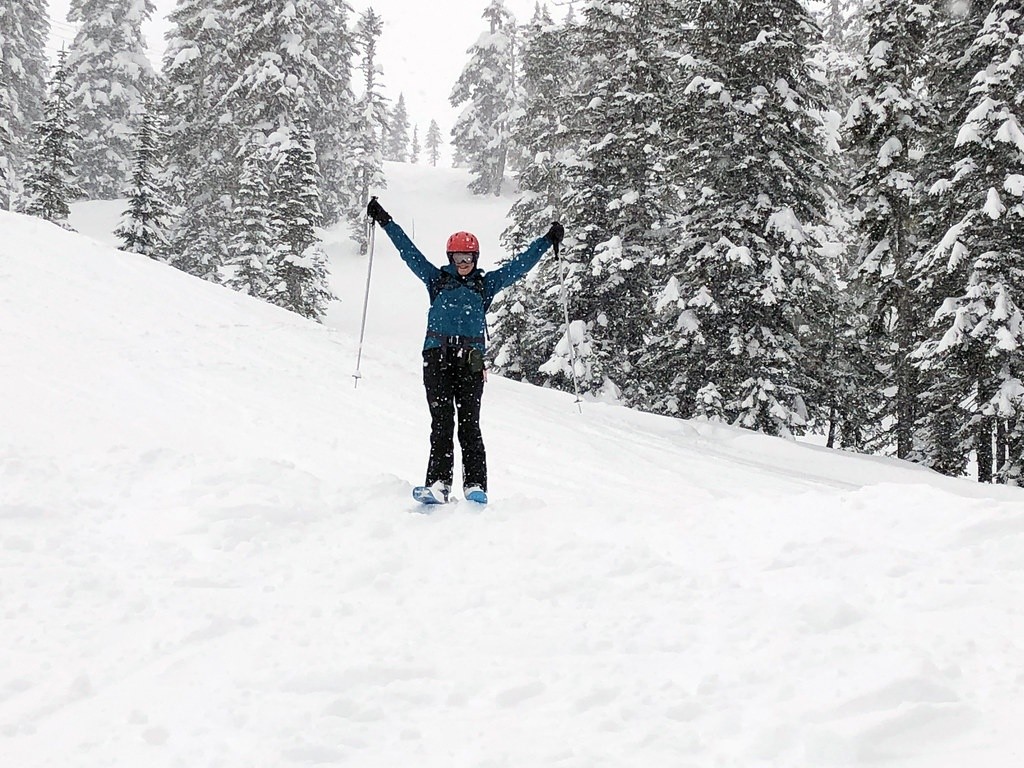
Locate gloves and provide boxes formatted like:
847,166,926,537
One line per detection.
546,225,565,244
367,199,392,227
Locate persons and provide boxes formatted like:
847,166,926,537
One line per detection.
367,198,564,505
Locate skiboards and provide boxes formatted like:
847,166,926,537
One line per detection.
412,481,486,502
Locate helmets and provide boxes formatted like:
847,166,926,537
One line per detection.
446,231,479,255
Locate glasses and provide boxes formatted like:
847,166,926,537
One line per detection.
452,253,473,264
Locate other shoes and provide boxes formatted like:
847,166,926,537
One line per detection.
426,480,450,501
463,481,485,492
